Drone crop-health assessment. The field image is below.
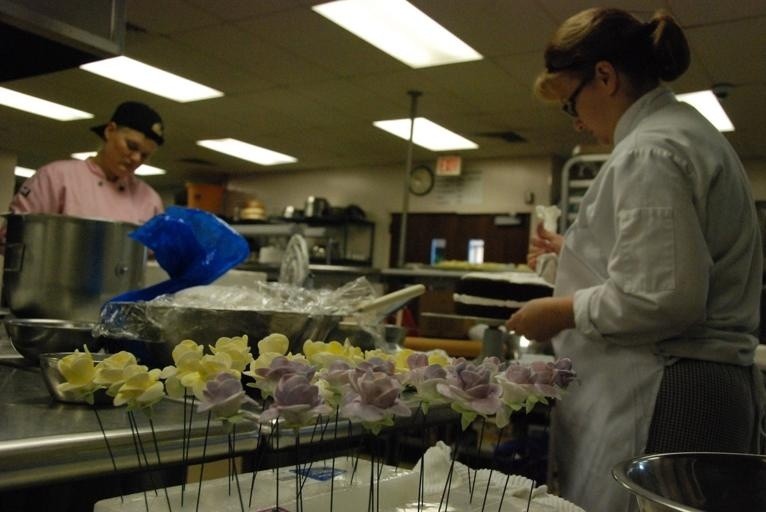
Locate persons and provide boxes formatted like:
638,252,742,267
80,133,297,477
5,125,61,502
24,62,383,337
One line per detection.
0,99,168,287
502,6,766,512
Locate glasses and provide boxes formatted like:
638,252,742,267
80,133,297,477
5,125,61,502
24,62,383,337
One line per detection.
560,76,592,119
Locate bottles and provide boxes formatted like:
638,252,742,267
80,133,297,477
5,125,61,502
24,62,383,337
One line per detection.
468,240,484,265
431,239,447,266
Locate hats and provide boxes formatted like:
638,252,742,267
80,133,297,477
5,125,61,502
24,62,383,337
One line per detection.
89,100,165,148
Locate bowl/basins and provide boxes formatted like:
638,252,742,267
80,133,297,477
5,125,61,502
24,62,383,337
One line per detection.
7,319,107,364
337,321,407,351
612,452,766,512
233,197,327,222
243,244,331,265
112,299,351,367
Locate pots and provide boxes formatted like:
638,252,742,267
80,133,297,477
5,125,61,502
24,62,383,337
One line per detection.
1,212,148,318
